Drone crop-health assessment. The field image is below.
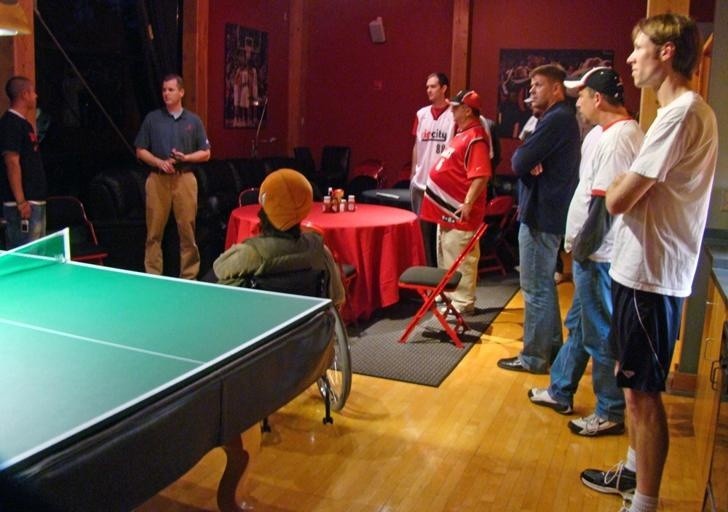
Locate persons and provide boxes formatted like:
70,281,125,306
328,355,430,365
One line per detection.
418,89,492,317
527,67,646,437
1,77,48,250
518,91,538,142
135,74,210,280
410,71,461,303
497,64,581,375
233,39,259,128
554,252,564,285
581,10,718,511
213,168,345,310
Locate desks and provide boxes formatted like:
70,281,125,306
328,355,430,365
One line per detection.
225,201,427,338
1,239,341,512
360,188,412,209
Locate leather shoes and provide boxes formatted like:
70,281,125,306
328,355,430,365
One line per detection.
498,357,527,371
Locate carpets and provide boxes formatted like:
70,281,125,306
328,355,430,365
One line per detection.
321,266,521,389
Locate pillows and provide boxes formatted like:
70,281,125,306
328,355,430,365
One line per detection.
292,147,316,174
317,145,350,180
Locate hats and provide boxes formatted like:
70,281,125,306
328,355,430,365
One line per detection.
562,66,624,101
258,168,313,232
449,89,480,110
523,93,533,103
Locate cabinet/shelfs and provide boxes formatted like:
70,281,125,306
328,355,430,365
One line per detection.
687,272,728,511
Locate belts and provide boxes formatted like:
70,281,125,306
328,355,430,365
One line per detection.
154,168,191,175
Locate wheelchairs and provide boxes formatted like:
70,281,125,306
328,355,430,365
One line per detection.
238,270,351,433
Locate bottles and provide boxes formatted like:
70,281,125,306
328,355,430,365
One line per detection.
321,187,356,213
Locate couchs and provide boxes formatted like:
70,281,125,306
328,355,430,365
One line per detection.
484,172,522,272
85,144,377,275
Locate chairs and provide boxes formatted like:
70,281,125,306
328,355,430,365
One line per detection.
477,192,518,280
397,223,487,350
42,196,110,267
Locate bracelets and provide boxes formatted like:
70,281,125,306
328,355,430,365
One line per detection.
15,198,26,206
463,199,474,206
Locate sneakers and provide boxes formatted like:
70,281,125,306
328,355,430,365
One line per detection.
579,460,635,502
434,294,450,302
567,412,625,436
553,272,563,284
527,387,572,415
440,307,474,316
